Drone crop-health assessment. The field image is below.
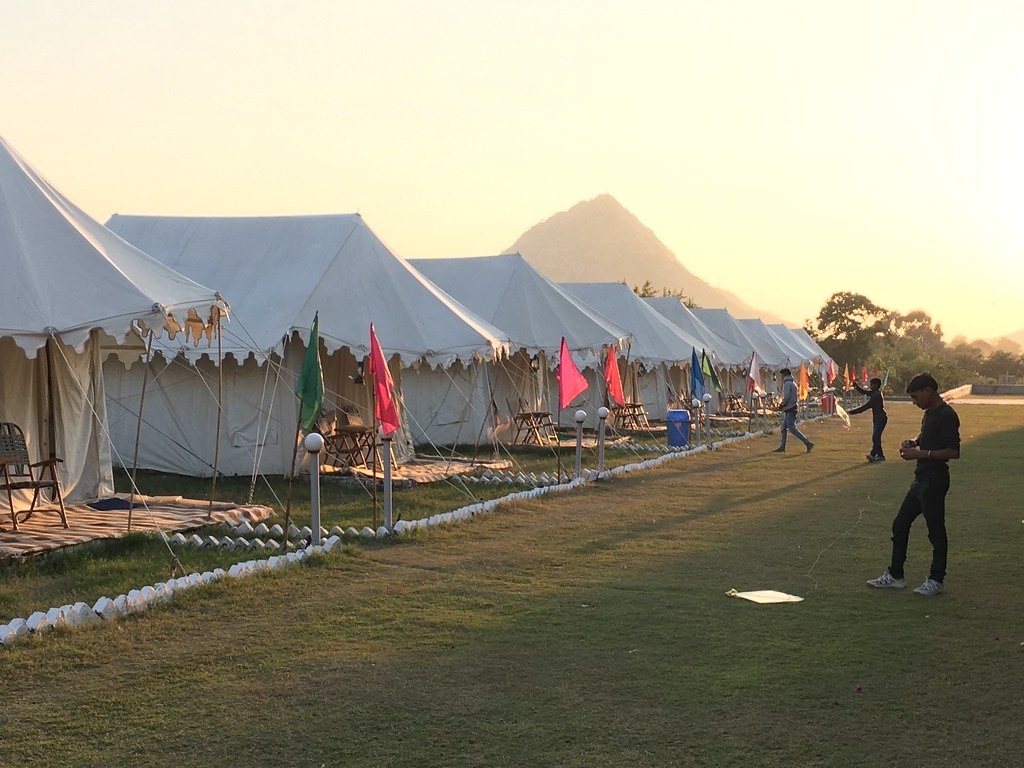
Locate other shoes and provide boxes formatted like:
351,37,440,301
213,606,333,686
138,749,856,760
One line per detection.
865,454,874,462
773,448,785,452
875,456,885,461
806,442,815,453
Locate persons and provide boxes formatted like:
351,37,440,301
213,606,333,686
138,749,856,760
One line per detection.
865,371,962,596
774,369,815,453
848,377,888,461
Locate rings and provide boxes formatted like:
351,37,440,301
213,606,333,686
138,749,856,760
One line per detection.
901,452,904,456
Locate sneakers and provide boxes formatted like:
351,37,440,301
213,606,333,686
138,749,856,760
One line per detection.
866,571,905,589
913,576,943,596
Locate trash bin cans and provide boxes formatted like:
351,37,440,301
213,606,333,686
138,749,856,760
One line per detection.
821,394,836,414
664,409,692,446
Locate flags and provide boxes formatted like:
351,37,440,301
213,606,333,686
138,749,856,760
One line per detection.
367,327,401,435
691,350,705,400
747,354,755,391
605,347,625,408
294,313,323,431
702,351,723,392
557,337,589,409
820,360,878,392
798,363,808,401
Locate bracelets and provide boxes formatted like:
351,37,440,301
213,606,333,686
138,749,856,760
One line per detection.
927,450,932,457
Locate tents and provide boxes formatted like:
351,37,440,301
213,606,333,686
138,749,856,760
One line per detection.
404,257,633,444
555,279,709,430
103,213,509,475
1,133,227,515
643,296,839,414
